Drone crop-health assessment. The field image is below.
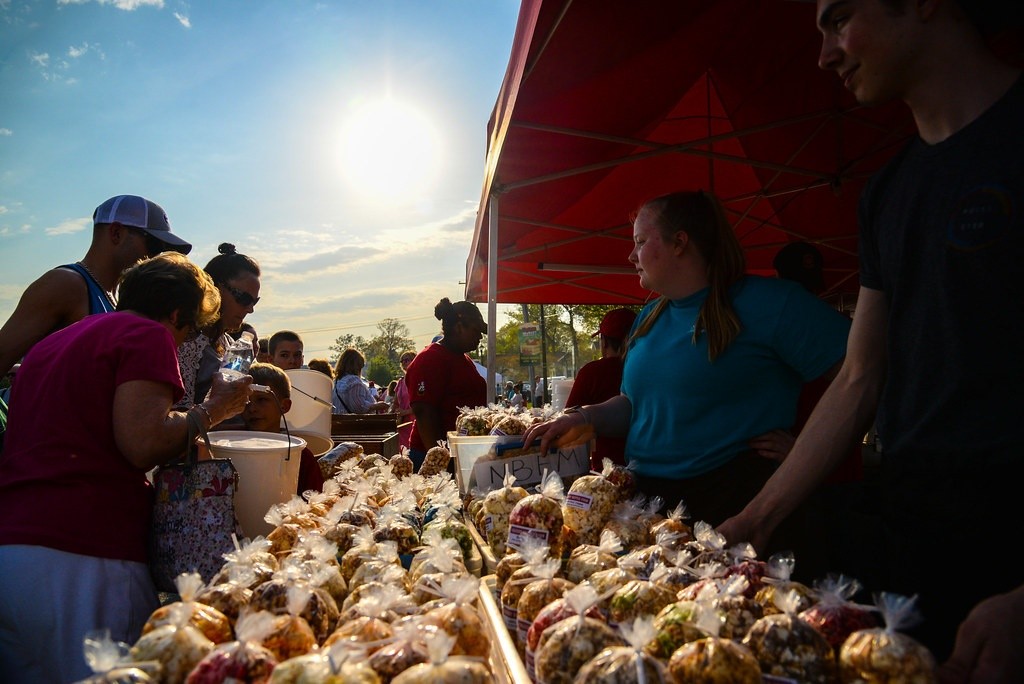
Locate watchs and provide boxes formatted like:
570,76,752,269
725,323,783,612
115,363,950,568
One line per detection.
565,405,591,428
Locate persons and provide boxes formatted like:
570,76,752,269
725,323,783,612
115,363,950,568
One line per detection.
494,376,544,408
710,1,1024,684
405,296,486,474
520,188,853,591
0,195,321,684
772,240,867,587
331,348,418,454
567,308,638,473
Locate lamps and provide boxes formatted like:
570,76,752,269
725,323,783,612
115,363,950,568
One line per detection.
537,245,638,275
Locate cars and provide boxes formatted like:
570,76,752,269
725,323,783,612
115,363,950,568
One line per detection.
521,376,567,406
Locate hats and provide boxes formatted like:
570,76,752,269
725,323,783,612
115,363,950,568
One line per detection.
454,301,488,334
591,308,637,340
92,194,192,256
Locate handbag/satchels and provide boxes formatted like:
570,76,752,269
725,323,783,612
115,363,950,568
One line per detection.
149,410,242,591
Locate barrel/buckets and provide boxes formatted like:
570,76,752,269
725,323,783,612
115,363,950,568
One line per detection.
278,368,336,457
551,379,575,413
195,383,307,542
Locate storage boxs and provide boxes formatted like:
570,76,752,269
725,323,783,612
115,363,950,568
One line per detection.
447,431,591,497
331,413,399,459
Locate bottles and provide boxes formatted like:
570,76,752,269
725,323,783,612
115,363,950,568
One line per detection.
204,330,254,403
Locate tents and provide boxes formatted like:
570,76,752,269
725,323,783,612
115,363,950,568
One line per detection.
464,0,921,412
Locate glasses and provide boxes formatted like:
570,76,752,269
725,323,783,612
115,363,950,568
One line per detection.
132,228,162,260
216,279,261,311
184,318,202,342
400,358,412,363
